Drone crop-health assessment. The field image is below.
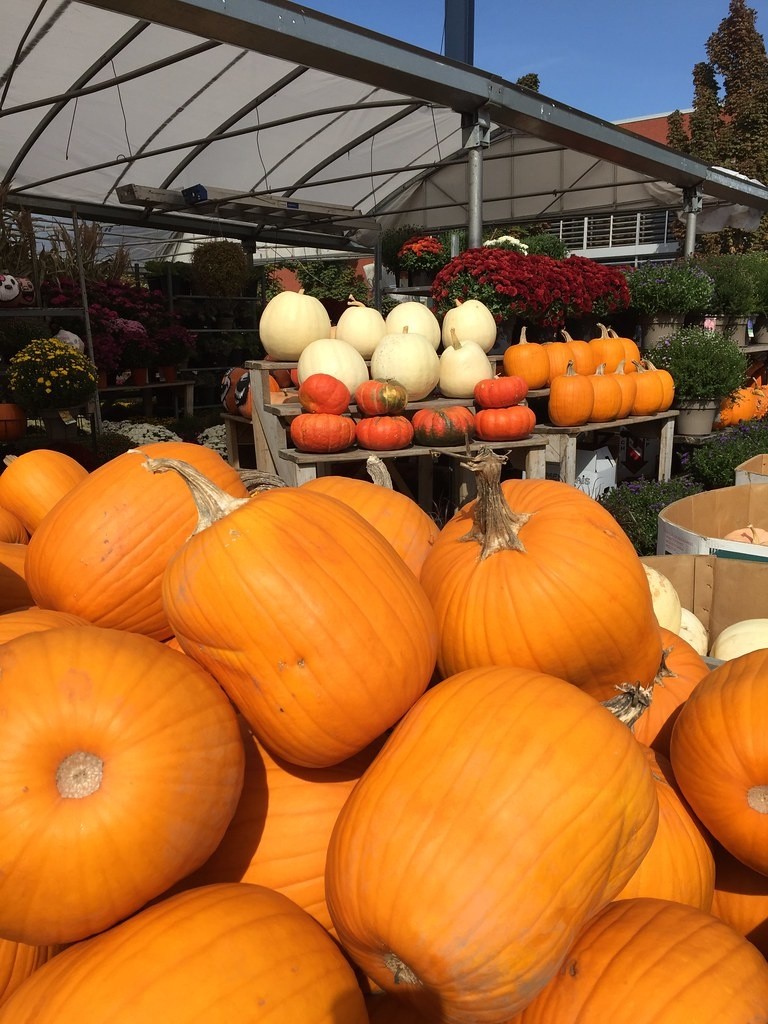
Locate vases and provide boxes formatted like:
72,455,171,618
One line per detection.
158,366,176,385
38,405,82,440
133,368,150,385
675,396,720,436
0,404,26,440
642,311,686,350
488,316,516,354
560,317,588,339
754,314,768,343
704,313,750,348
411,267,438,286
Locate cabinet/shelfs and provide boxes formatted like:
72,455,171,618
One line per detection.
220,355,680,489
166,265,268,420
0,307,99,467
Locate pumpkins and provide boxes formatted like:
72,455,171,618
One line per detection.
711,374,768,430
259,286,674,452
0,441,768,1024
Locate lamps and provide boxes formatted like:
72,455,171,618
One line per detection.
181,183,208,205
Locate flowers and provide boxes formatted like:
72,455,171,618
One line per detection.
380,223,768,556
6,338,100,409
103,396,228,463
40,276,198,386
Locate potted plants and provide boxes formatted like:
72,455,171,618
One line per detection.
146,240,369,407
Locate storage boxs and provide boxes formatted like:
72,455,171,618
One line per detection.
612,434,660,482
639,453,768,653
521,443,617,502
